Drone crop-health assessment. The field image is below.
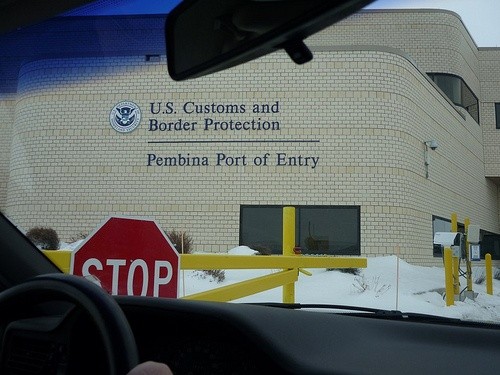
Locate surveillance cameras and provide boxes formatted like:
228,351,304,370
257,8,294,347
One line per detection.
430,140,437,150
433,231,460,246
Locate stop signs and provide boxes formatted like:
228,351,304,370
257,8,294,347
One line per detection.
68,215,181,300
